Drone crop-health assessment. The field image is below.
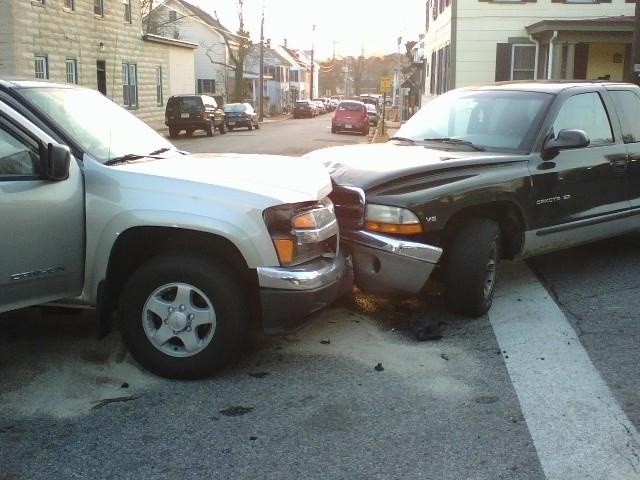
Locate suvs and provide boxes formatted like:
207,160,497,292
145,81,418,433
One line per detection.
0,78,355,380
298,77,640,320
163,94,229,139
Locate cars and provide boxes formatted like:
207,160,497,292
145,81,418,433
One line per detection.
330,101,370,136
357,93,392,127
289,93,347,120
222,101,261,131
